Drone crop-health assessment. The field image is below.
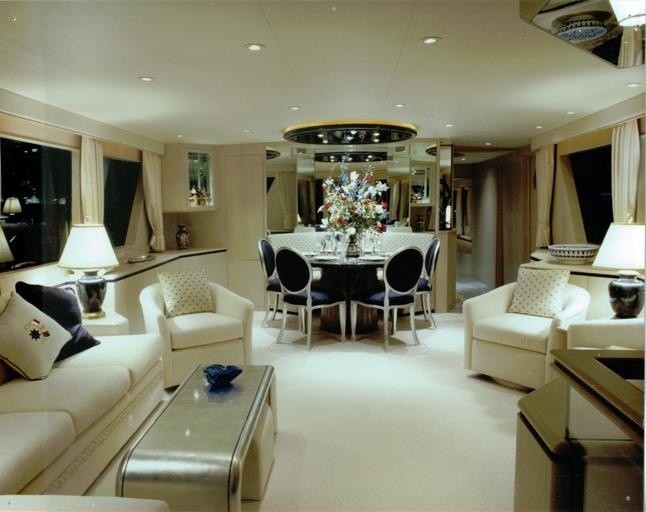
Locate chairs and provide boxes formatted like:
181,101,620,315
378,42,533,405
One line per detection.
258,239,282,326
348,245,424,351
417,238,441,329
274,246,346,350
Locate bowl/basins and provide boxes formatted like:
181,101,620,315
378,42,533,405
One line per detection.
549,243,599,264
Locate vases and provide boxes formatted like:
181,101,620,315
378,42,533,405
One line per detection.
346,235,360,256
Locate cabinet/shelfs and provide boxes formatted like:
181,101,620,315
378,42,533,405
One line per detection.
409,138,439,234
104,247,228,335
162,142,222,212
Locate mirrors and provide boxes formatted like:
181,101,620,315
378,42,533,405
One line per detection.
440,145,452,230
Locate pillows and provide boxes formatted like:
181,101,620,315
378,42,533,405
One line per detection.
15,282,101,363
0,291,73,380
507,267,570,318
157,270,216,316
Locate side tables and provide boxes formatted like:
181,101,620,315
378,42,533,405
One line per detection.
568,313,646,439
80,310,130,335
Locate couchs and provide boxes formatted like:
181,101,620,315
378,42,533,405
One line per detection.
463,264,592,390
140,268,254,389
0,281,170,512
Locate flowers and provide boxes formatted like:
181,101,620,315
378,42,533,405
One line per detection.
317,162,390,241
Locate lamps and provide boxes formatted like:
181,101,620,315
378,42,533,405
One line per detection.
57,223,118,319
313,151,387,163
4,197,23,223
282,121,419,145
609,0,646,27
